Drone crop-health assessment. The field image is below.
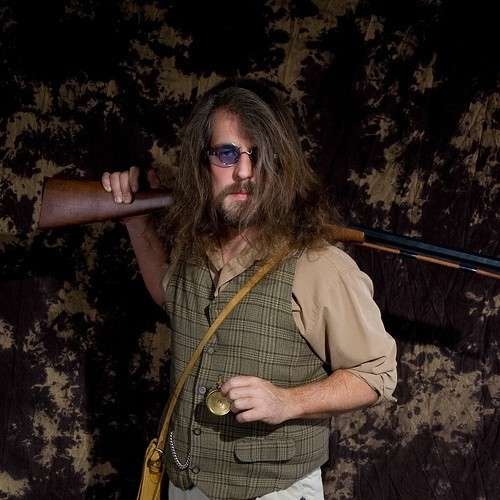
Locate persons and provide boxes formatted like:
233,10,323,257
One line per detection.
103,76,397,500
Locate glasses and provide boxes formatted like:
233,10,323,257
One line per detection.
206,143,279,168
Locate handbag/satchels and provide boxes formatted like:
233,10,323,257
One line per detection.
137,437,170,500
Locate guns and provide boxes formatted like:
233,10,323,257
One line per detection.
36,176,500,279
